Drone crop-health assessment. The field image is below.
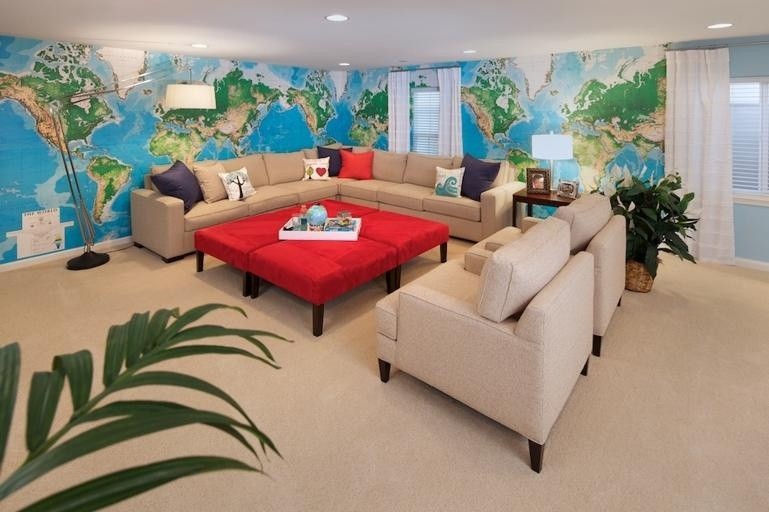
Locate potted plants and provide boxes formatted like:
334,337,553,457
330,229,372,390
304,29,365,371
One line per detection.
602,168,701,293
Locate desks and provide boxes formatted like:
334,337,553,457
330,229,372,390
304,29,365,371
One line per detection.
193,198,450,336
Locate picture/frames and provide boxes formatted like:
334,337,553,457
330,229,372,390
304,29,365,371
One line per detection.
557,179,579,200
526,168,551,194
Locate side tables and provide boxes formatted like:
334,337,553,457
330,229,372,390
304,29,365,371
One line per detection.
511,187,575,228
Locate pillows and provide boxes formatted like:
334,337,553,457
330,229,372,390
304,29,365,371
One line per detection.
459,152,501,202
316,145,352,177
301,156,328,181
148,159,202,215
192,163,227,204
433,166,465,199
218,166,256,201
339,149,375,180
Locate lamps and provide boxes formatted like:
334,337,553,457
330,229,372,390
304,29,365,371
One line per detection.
50,63,217,272
531,130,573,194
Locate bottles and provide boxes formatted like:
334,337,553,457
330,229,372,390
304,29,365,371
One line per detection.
299,205,308,231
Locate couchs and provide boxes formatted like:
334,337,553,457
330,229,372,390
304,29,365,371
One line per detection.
342,139,527,241
130,138,342,263
374,215,595,473
465,190,626,358
